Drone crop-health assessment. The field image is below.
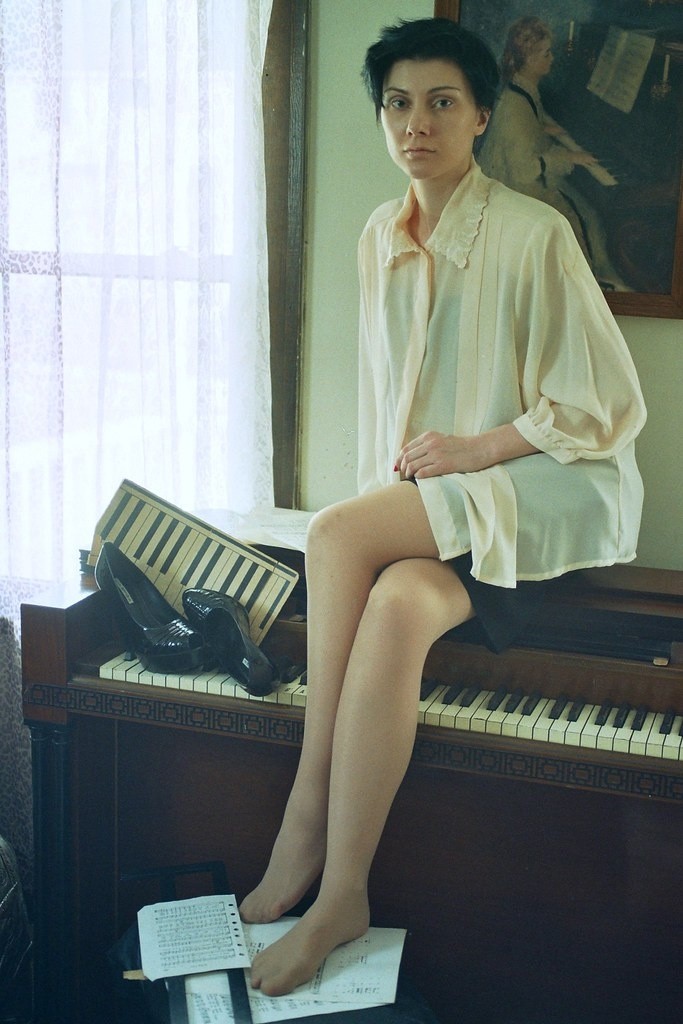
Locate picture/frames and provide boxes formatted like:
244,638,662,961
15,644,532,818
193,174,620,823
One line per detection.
433,0,683,320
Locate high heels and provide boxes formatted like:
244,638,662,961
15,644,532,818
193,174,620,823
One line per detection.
181,588,281,697
95,542,206,674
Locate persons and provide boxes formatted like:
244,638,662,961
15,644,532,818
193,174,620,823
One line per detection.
237,13,650,1000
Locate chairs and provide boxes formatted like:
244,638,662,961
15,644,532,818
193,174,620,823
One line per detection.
0,832,438,1024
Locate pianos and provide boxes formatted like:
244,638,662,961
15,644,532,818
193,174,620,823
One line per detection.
21,505,683,1023
